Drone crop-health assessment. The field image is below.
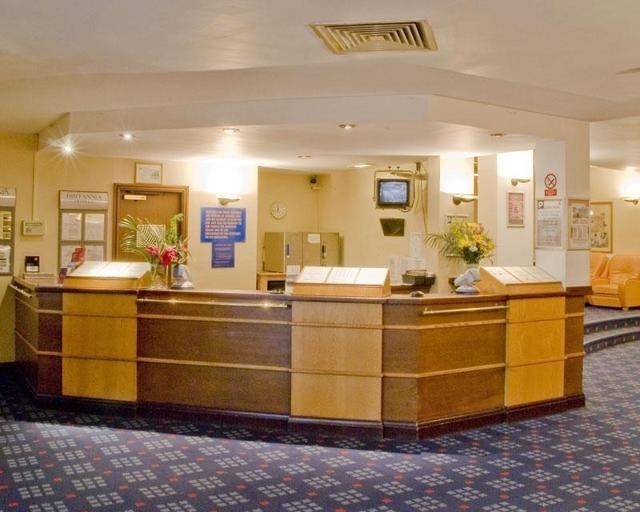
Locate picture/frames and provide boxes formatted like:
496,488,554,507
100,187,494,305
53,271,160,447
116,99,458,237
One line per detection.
590,200,613,254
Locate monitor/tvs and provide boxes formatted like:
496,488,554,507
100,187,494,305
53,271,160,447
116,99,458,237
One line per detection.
378,179,409,206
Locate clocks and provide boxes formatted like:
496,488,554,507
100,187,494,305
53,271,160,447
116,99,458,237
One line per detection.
270,200,287,220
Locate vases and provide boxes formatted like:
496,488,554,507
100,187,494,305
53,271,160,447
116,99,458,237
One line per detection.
467,264,480,272
147,264,168,291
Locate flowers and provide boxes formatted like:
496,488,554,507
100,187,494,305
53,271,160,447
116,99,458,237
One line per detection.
118,213,193,269
422,217,495,265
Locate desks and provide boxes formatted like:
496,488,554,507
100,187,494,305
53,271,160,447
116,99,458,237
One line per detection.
390,284,431,294
257,272,286,290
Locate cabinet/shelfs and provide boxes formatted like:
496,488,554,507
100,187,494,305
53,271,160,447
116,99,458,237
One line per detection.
263,232,341,273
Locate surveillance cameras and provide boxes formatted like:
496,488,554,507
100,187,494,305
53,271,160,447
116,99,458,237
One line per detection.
310,178,320,190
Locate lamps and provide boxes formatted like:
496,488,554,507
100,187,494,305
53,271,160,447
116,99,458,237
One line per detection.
440,168,479,205
512,178,531,187
624,198,638,206
219,197,240,205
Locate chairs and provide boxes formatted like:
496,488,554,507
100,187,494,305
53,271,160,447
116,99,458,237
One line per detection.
585,253,609,304
588,253,640,312
